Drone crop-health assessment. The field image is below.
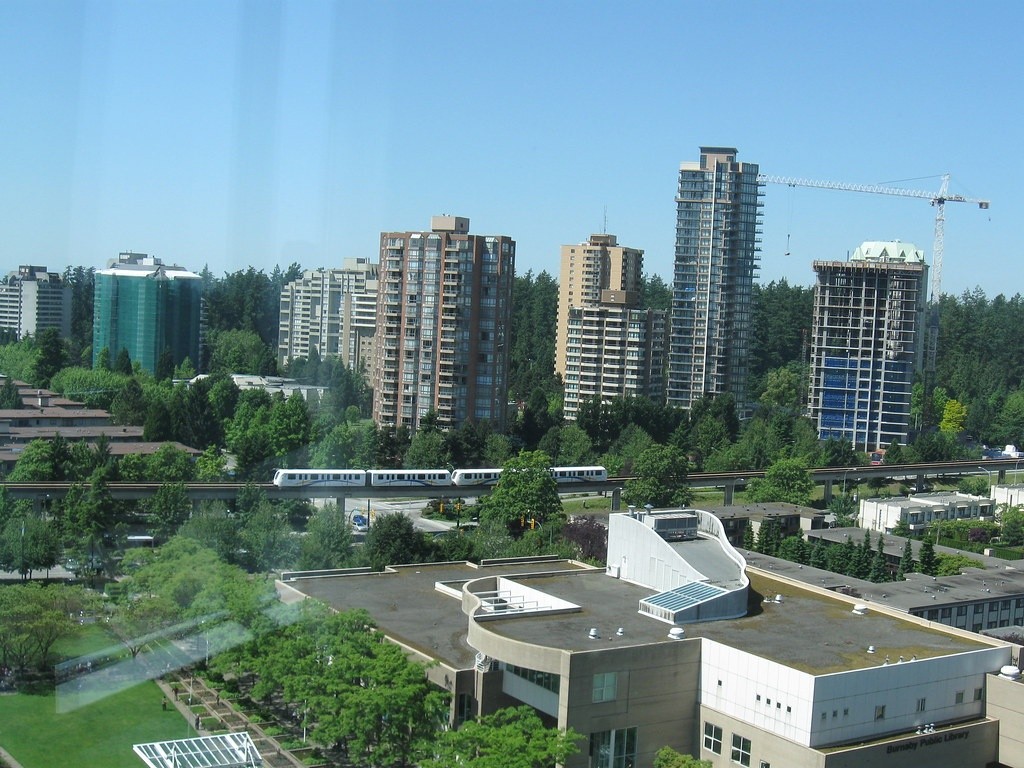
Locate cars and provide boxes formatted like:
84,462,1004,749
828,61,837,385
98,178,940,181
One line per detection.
353,515,366,524
352,522,368,532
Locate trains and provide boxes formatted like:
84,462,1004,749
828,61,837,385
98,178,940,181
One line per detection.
273,466,607,487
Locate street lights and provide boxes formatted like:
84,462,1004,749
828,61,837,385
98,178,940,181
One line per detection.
843,468,857,499
610,487,623,511
978,466,990,491
1015,459,1024,484
732,478,744,506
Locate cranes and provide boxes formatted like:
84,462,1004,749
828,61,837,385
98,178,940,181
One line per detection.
754,173,990,434
